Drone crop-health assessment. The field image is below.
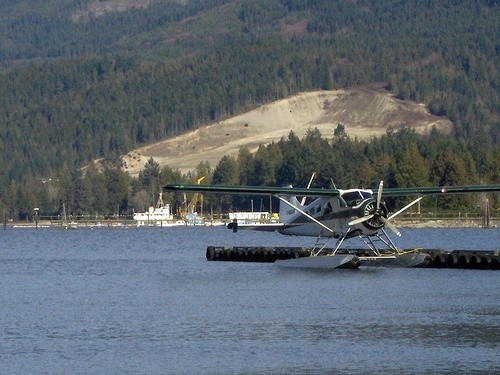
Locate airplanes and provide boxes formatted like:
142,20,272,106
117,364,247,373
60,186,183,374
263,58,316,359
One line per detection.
160,172,499,269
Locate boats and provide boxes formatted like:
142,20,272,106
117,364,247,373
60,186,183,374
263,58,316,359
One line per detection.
134,193,170,221
